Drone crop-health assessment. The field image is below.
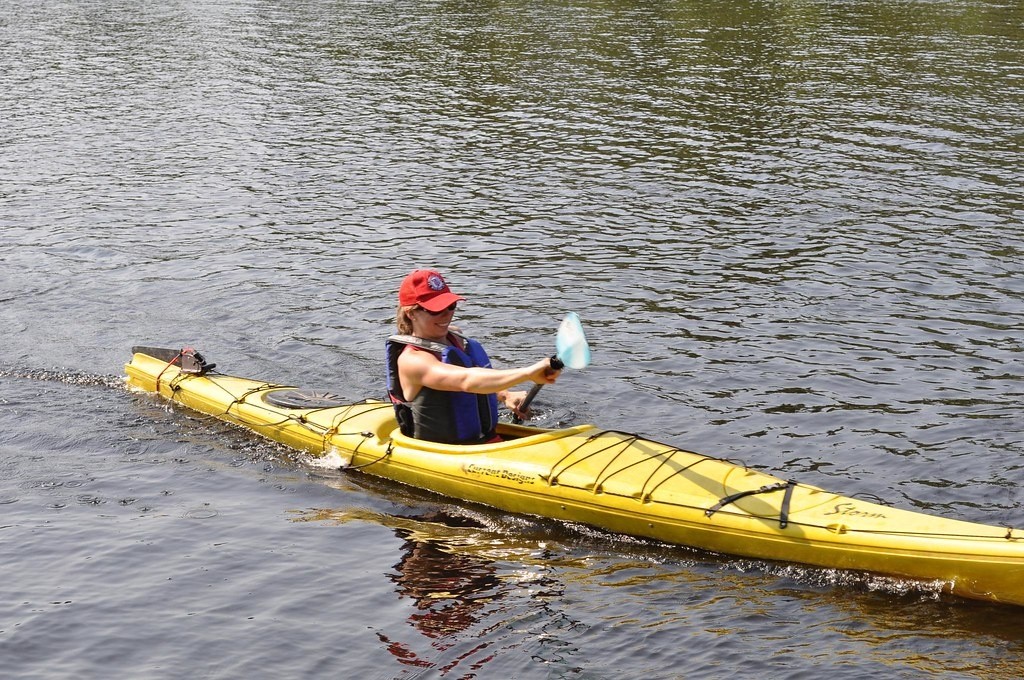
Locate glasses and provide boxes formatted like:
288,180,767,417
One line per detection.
417,302,456,316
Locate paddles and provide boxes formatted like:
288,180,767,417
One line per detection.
512,309,592,427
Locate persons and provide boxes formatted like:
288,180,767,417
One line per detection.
385,270,563,444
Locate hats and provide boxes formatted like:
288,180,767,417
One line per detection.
398,269,467,313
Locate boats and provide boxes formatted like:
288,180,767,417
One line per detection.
125,346,1024,607
